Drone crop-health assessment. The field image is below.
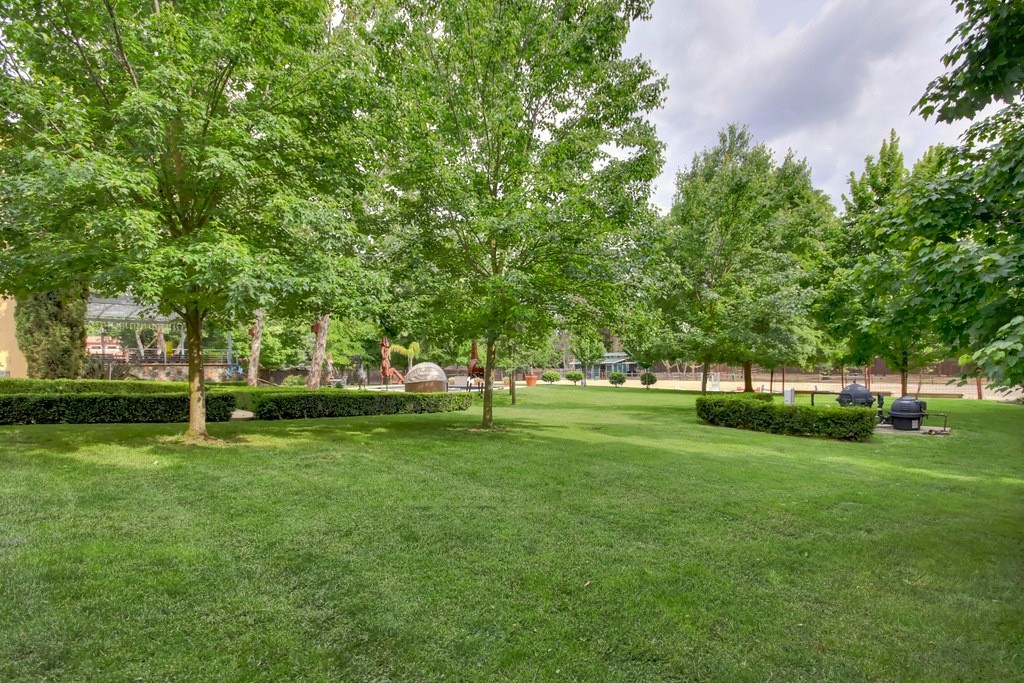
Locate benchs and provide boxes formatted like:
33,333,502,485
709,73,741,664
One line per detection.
871,391,894,397
907,392,964,398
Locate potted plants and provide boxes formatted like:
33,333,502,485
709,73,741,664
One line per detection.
449,376,455,385
501,376,509,383
